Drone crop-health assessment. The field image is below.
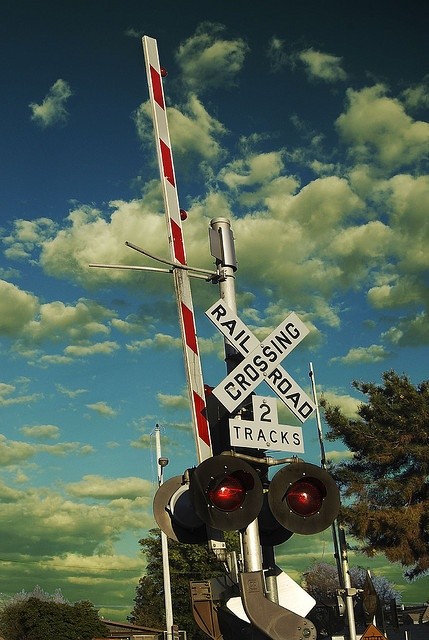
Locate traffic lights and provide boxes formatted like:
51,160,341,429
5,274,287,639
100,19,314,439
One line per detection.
191,455,340,535
152,476,292,545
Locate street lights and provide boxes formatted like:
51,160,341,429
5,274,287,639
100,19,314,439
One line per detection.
156,425,173,640
